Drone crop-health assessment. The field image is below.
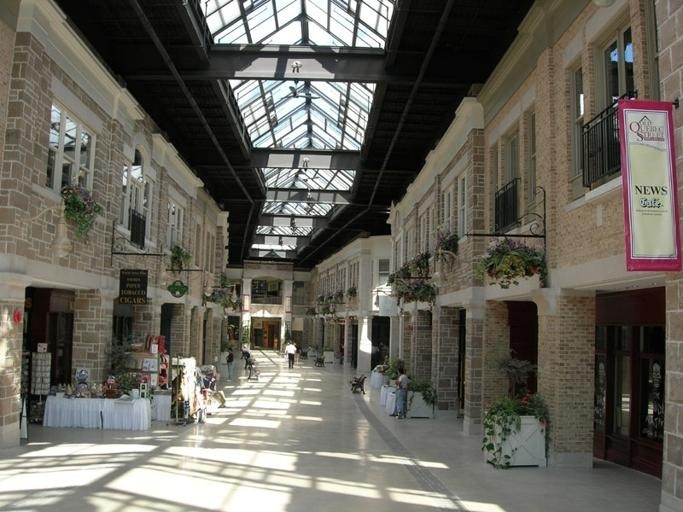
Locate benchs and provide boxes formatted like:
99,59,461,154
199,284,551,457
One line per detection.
314,356,325,367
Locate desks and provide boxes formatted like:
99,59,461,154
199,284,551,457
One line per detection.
44,393,151,431
154,394,173,421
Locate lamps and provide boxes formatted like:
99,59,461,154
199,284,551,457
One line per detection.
374,249,488,308
21,203,238,303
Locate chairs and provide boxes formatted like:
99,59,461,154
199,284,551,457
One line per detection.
349,374,367,393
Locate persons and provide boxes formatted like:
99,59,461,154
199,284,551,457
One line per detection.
395,366,410,420
224,347,234,382
284,340,297,369
388,375,399,416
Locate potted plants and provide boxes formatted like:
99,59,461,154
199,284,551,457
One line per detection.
484,393,549,472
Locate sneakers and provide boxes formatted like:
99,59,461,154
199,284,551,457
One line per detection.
389,414,405,419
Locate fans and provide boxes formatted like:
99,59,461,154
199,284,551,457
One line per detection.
280,79,320,100
